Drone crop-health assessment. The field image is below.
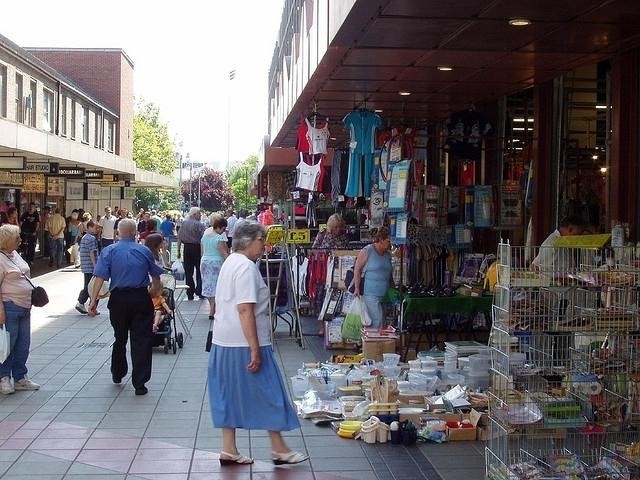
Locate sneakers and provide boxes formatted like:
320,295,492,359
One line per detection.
0,376,15,394
14,379,40,389
87,305,100,315
75,302,88,314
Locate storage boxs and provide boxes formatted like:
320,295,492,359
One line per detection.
290,335,572,444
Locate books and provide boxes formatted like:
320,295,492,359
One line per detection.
494,268,640,480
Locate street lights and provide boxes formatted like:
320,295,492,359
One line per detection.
226,67,238,172
186,152,193,209
197,169,208,207
175,151,182,213
244,165,249,212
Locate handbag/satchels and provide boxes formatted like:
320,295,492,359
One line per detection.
32,286,48,307
88,276,109,299
344,270,364,295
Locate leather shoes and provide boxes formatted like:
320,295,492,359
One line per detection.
112,374,121,383
135,387,148,395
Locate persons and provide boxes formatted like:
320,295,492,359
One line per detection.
310,215,350,336
2,203,66,269
176,206,261,320
210,220,309,466
528,216,584,311
0,224,40,396
352,227,394,329
67,205,184,396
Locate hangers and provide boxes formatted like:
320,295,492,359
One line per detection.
351,102,374,114
303,103,330,124
292,241,451,257
334,144,351,152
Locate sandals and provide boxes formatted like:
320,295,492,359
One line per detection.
272,451,308,465
219,451,254,465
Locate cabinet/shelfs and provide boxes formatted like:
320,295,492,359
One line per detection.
484,240,639,480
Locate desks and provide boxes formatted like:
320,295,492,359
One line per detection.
390,285,495,362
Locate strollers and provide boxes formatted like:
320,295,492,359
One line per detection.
148,266,184,355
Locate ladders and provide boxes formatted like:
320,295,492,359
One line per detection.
256,224,306,349
